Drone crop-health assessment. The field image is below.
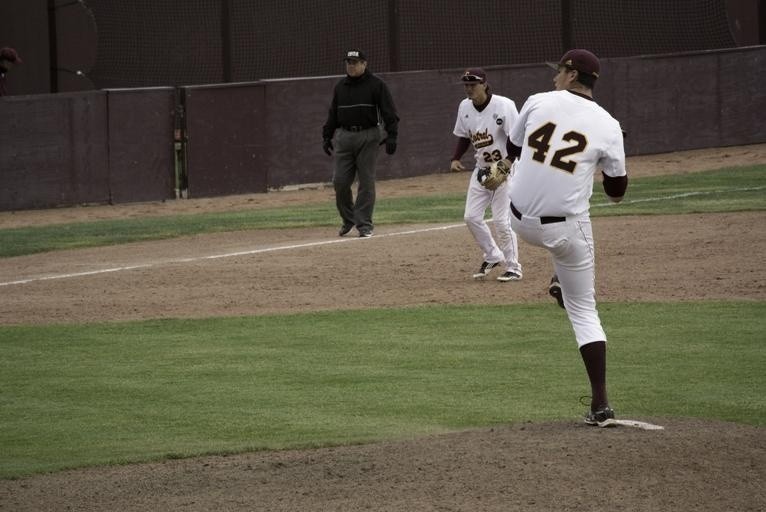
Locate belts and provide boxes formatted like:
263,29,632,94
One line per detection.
340,123,377,133
510,201,566,225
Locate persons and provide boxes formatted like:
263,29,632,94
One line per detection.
506,48,629,429
321,49,400,238
449,65,523,283
0,47,25,98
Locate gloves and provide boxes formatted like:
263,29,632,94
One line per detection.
378,135,398,154
322,137,333,156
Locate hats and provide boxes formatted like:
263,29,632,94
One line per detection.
545,48,601,81
460,67,488,84
342,47,368,62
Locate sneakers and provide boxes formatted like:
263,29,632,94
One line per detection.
497,271,522,281
359,228,374,237
338,222,355,236
579,409,616,428
549,277,565,310
472,260,499,280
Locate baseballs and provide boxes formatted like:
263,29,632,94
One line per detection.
481,175,487,183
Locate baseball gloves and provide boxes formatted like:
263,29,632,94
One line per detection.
478,160,511,190
